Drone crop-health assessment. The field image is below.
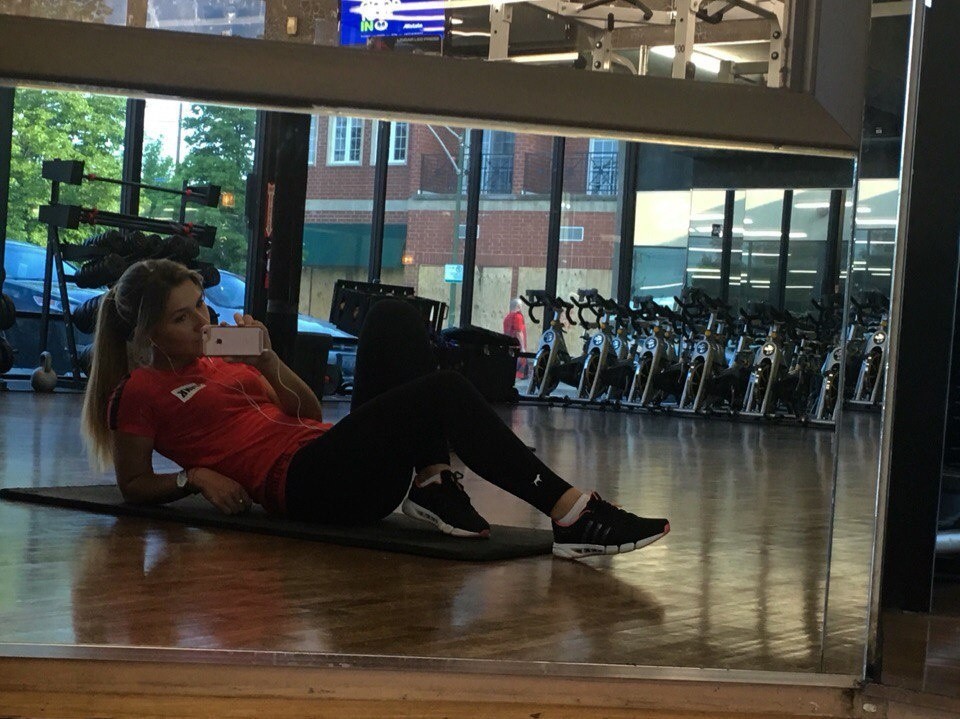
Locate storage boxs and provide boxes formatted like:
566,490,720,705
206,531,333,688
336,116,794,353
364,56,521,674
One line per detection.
330,278,443,339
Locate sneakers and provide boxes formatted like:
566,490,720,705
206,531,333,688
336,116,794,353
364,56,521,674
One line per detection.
551,490,671,559
402,470,490,537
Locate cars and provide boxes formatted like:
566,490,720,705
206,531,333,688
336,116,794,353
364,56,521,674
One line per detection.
204,270,358,391
4,239,113,376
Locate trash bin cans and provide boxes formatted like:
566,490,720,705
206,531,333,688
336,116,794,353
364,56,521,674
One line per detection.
296,332,336,402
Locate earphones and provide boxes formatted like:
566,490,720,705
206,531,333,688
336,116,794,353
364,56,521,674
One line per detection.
147,337,158,347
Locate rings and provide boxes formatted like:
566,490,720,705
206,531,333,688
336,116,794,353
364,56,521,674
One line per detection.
238,499,244,505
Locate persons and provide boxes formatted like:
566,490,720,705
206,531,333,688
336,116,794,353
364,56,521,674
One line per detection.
81,258,671,558
503,298,529,380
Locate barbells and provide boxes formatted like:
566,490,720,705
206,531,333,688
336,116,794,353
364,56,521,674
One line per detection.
0,293,20,374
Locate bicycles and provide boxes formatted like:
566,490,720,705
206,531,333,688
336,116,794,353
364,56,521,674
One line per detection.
519,285,895,415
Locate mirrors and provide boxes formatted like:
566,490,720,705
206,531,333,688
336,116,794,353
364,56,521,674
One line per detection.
0,77,858,690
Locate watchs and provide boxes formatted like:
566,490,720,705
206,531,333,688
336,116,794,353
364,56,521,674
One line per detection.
176,470,188,499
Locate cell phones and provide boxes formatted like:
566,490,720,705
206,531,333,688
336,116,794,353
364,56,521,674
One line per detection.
201,324,263,356
